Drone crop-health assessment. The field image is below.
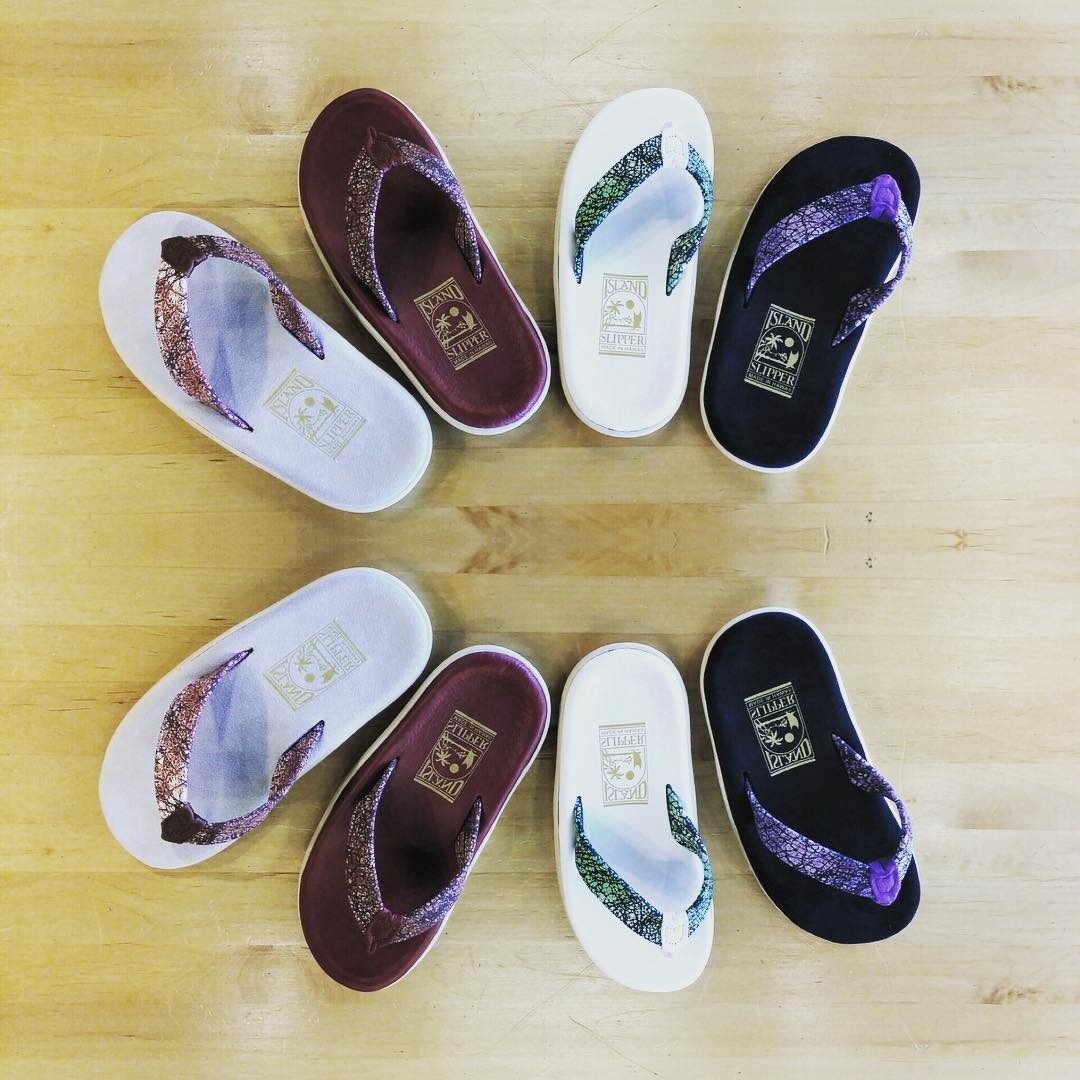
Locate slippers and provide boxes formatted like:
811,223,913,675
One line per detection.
698,608,922,943
299,643,552,992
699,134,921,477
295,88,552,435
555,88,715,439
554,642,713,995
99,208,433,514
97,567,433,871
